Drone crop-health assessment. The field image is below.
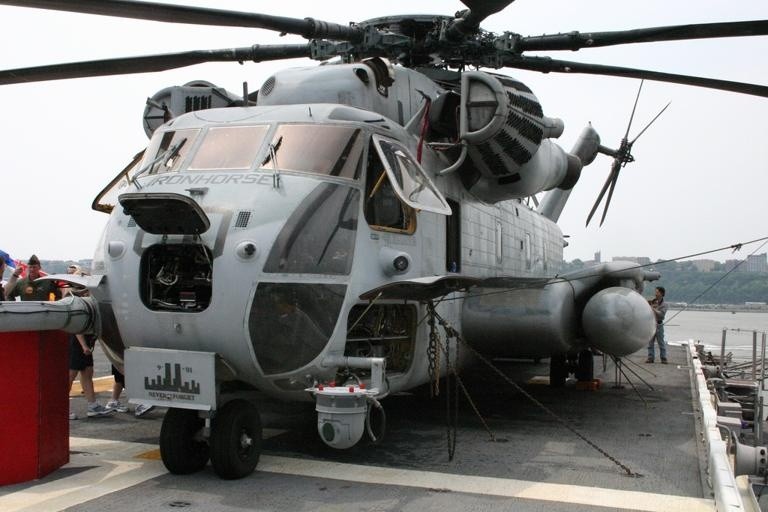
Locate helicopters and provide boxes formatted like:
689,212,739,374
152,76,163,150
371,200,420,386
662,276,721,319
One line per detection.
0,0,767,480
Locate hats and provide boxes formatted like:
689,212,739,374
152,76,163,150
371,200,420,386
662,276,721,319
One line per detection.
27,254,41,266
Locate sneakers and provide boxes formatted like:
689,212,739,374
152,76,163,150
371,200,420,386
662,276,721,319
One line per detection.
661,358,668,364
134,402,156,418
69,411,76,420
645,359,654,363
106,400,130,413
86,404,115,418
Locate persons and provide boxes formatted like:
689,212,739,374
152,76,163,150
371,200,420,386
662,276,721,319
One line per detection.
0,248,156,420
645,286,669,364
